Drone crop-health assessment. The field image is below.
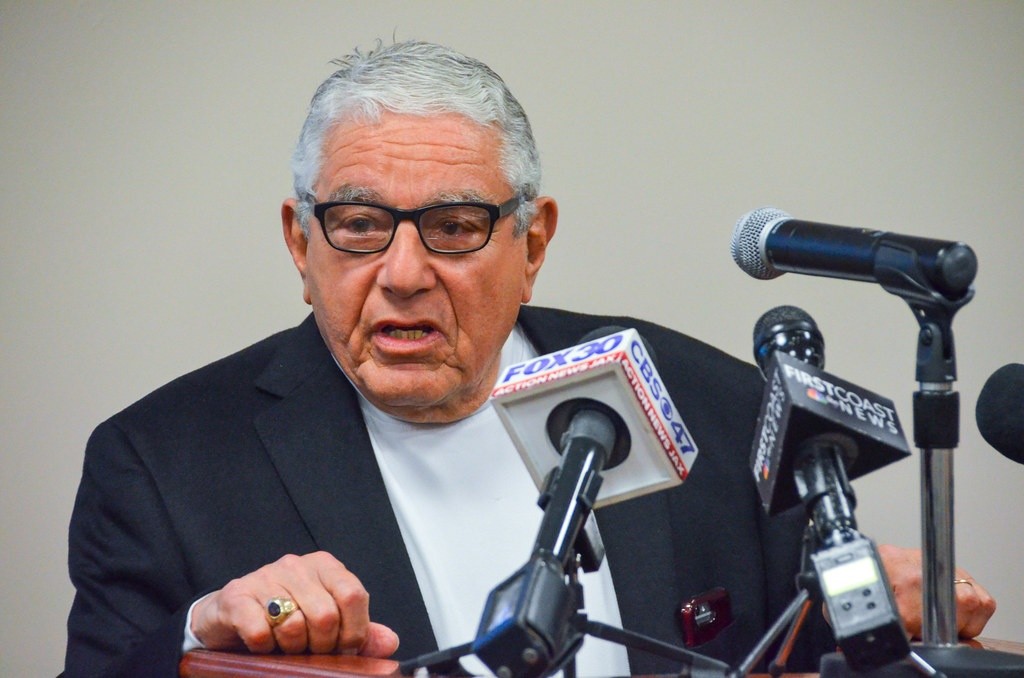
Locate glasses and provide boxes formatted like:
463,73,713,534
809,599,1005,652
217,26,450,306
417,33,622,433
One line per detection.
314,194,521,255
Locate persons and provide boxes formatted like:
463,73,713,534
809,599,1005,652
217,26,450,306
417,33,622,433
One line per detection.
55,27,998,676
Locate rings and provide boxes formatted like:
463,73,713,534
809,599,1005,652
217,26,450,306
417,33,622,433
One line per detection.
264,596,299,628
954,578,973,586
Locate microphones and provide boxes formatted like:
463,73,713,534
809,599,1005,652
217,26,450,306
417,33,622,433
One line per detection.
490,325,698,575
730,208,977,300
747,305,912,547
975,363,1024,467
